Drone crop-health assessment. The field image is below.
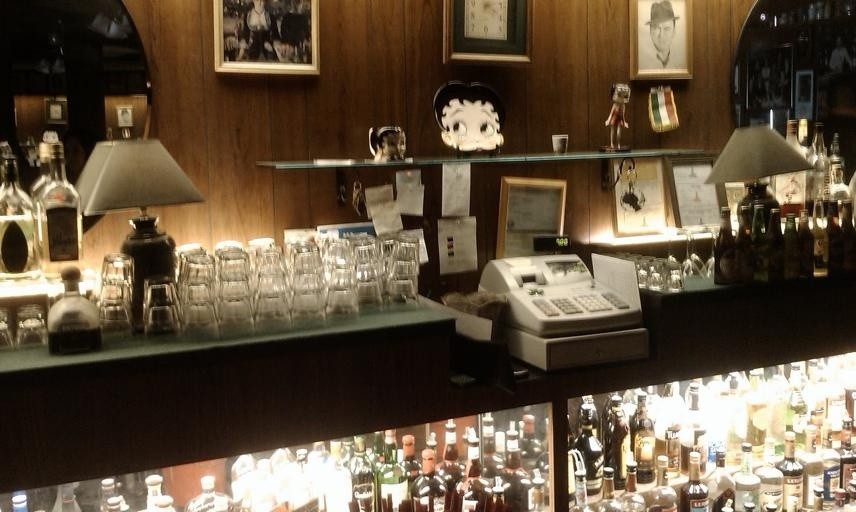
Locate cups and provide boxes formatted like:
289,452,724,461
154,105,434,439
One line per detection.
212,247,256,333
144,303,182,338
648,264,663,289
675,227,721,281
636,262,650,287
95,253,134,332
253,246,293,330
383,234,421,304
320,237,361,318
175,246,220,337
669,264,684,291
142,280,182,321
604,252,678,284
16,306,47,349
289,242,327,322
347,235,385,309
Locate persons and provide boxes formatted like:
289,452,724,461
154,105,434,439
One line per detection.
376,128,410,162
237,0,282,62
641,0,685,70
606,83,630,149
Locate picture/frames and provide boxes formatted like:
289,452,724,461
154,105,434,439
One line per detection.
629,0,693,80
608,153,677,239
443,0,536,67
496,176,569,262
667,157,726,229
213,0,319,76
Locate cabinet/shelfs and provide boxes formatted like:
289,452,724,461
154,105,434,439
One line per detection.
0,292,856,512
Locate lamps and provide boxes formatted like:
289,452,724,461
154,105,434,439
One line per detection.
701,127,813,236
75,137,205,314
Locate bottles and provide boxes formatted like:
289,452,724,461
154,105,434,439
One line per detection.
46,269,101,355
735,445,758,511
797,210,814,277
682,384,706,476
144,475,165,510
412,451,452,511
681,452,708,512
101,481,117,510
811,123,829,200
13,496,27,511
653,385,687,471
376,429,417,511
832,489,846,510
537,421,567,501
784,213,798,280
804,427,825,511
840,201,855,279
757,438,783,512
323,441,351,510
778,431,804,512
534,480,546,509
752,204,767,289
39,143,83,273
826,200,845,278
830,159,850,200
734,206,760,284
812,488,824,512
605,399,632,489
748,372,769,444
650,456,677,512
767,208,792,292
349,438,378,511
521,417,548,474
239,447,325,512
185,476,234,511
398,436,425,477
595,468,619,511
714,208,735,284
786,364,810,434
437,425,468,486
812,199,828,277
803,360,832,427
482,419,507,481
838,417,855,498
107,498,123,511
768,367,800,435
825,358,854,430
619,462,646,511
778,120,813,213
843,355,856,430
820,426,844,499
504,430,535,512
629,395,656,483
456,440,490,512
709,451,735,512
0,155,36,274
54,482,84,511
154,496,173,510
568,471,594,511
575,406,605,494
